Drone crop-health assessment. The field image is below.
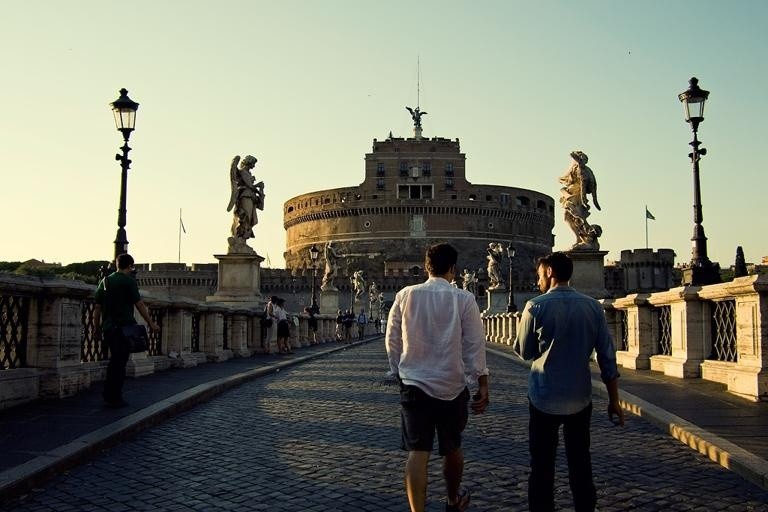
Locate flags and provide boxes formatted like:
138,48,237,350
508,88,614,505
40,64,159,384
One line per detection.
180,219,186,234
646,209,656,220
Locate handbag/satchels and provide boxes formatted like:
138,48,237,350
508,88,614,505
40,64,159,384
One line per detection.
261,318,273,328
122,323,150,353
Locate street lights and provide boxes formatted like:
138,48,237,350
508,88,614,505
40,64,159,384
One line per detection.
108,87,140,277
379,303,384,319
349,276,356,316
505,243,519,312
369,290,373,320
307,243,320,315
472,273,479,303
679,74,720,285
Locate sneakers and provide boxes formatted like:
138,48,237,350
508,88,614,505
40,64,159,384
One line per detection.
446,484,470,511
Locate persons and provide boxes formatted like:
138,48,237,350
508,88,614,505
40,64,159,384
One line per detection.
304,307,322,345
343,309,353,343
460,268,473,290
378,288,385,307
486,243,506,288
94,254,161,404
384,243,489,511
368,281,378,304
310,243,319,267
353,270,367,300
227,154,265,241
336,309,344,343
264,296,278,355
274,298,296,354
358,308,367,340
553,150,599,249
320,240,347,291
513,252,625,512
